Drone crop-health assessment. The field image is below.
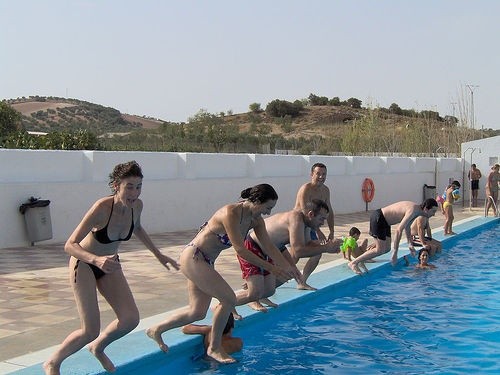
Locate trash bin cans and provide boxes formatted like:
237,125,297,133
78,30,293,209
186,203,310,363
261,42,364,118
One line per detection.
18,196,54,246
424,183,436,200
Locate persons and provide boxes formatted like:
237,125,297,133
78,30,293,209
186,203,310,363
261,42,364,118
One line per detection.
146,183,302,363
410,216,442,256
348,198,438,274
486,164,500,216
43,160,181,375
285,163,334,290
404,248,435,270
468,164,481,207
212,199,341,320
439,181,461,235
182,312,243,358
342,227,376,263
242,240,321,311
